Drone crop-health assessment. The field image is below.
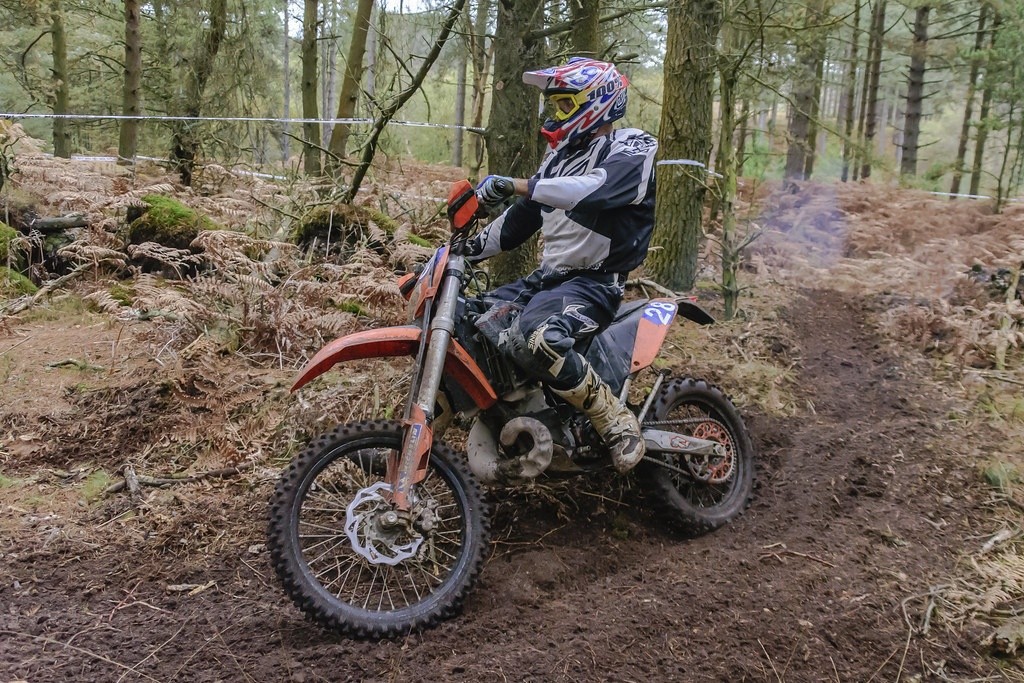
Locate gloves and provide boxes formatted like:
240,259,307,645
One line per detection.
476,176,516,214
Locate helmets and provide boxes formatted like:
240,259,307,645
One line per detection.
522,57,629,152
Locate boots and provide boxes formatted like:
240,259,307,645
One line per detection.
549,363,646,476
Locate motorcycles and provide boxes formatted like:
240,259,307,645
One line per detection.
267,178,755,640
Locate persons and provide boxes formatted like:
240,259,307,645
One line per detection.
449,57,658,474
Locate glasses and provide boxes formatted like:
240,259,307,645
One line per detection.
548,94,581,122
